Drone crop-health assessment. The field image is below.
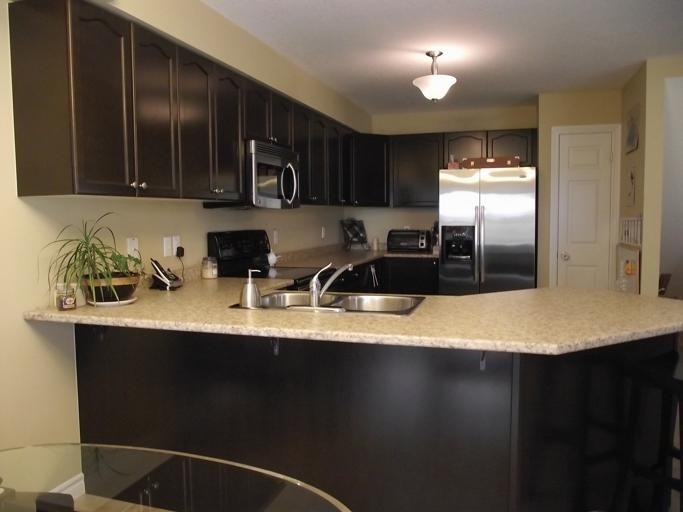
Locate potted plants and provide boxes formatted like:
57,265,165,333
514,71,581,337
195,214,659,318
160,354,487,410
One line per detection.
37,212,147,305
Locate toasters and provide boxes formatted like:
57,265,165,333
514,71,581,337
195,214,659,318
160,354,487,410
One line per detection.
385,228,431,252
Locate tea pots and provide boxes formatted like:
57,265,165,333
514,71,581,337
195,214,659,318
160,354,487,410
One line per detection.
267,248,282,265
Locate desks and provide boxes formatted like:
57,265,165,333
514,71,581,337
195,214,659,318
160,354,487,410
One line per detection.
1,442,351,511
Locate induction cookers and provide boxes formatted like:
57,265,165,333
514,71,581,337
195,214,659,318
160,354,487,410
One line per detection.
237,265,336,289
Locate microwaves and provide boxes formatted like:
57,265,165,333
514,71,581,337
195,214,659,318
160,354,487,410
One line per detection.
201,139,301,210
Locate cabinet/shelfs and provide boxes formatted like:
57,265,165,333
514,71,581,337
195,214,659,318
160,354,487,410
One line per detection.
9,0,177,199
247,73,357,208
384,255,439,297
389,133,444,208
444,126,538,168
352,131,389,207
179,38,246,207
334,258,382,293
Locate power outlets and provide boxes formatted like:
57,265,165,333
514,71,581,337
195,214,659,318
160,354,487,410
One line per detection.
162,235,181,257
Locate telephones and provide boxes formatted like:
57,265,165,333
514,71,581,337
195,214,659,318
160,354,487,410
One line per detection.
149,258,183,291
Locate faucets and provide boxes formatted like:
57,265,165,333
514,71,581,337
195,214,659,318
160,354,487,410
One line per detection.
307,262,353,306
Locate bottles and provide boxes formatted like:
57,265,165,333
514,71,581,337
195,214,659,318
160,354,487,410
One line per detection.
56,289,77,310
372,237,379,251
200,257,218,278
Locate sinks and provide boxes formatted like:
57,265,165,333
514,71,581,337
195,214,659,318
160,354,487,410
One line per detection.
326,294,427,318
227,292,343,313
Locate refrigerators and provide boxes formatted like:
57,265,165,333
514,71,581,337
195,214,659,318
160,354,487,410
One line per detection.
437,167,536,296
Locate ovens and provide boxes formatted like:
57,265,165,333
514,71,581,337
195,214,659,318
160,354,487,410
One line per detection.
298,275,343,293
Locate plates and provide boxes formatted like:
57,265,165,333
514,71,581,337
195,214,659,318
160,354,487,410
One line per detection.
85,296,137,308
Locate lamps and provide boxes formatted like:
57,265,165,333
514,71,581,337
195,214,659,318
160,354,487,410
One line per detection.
414,51,457,103
